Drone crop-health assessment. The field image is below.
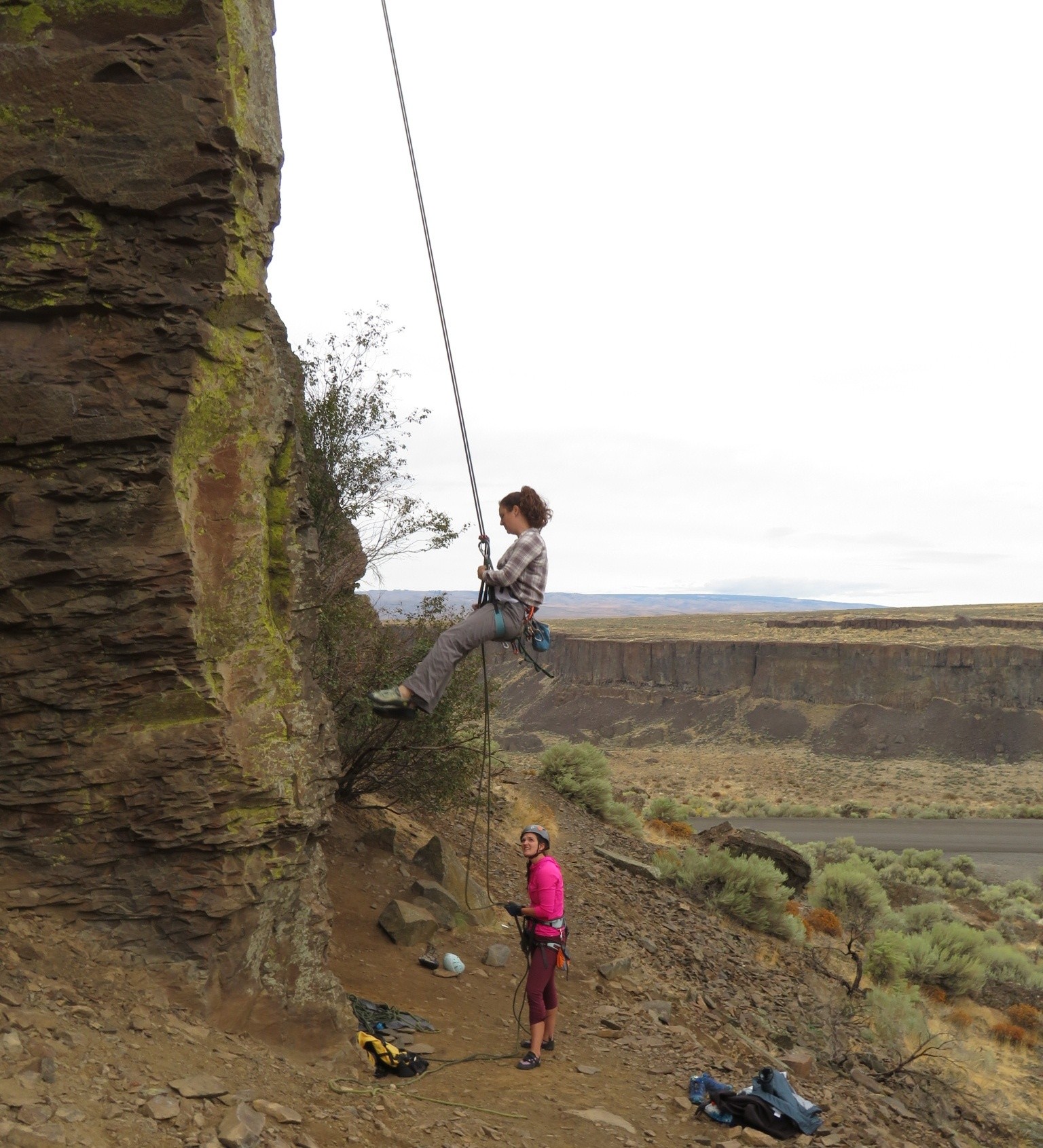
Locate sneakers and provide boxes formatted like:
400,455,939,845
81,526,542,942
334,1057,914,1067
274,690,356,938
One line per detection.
702,1074,732,1092
521,1039,554,1051
517,1051,541,1069
689,1076,705,1104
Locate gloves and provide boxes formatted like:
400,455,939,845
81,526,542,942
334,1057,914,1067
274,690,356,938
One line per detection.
504,901,526,916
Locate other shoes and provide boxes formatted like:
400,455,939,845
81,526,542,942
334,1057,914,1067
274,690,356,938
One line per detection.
368,685,408,707
372,704,417,721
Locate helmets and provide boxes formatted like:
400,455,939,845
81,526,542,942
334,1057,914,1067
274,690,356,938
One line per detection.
520,825,550,850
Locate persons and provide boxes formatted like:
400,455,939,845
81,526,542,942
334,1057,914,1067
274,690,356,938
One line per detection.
503,824,566,1070
365,485,553,719
